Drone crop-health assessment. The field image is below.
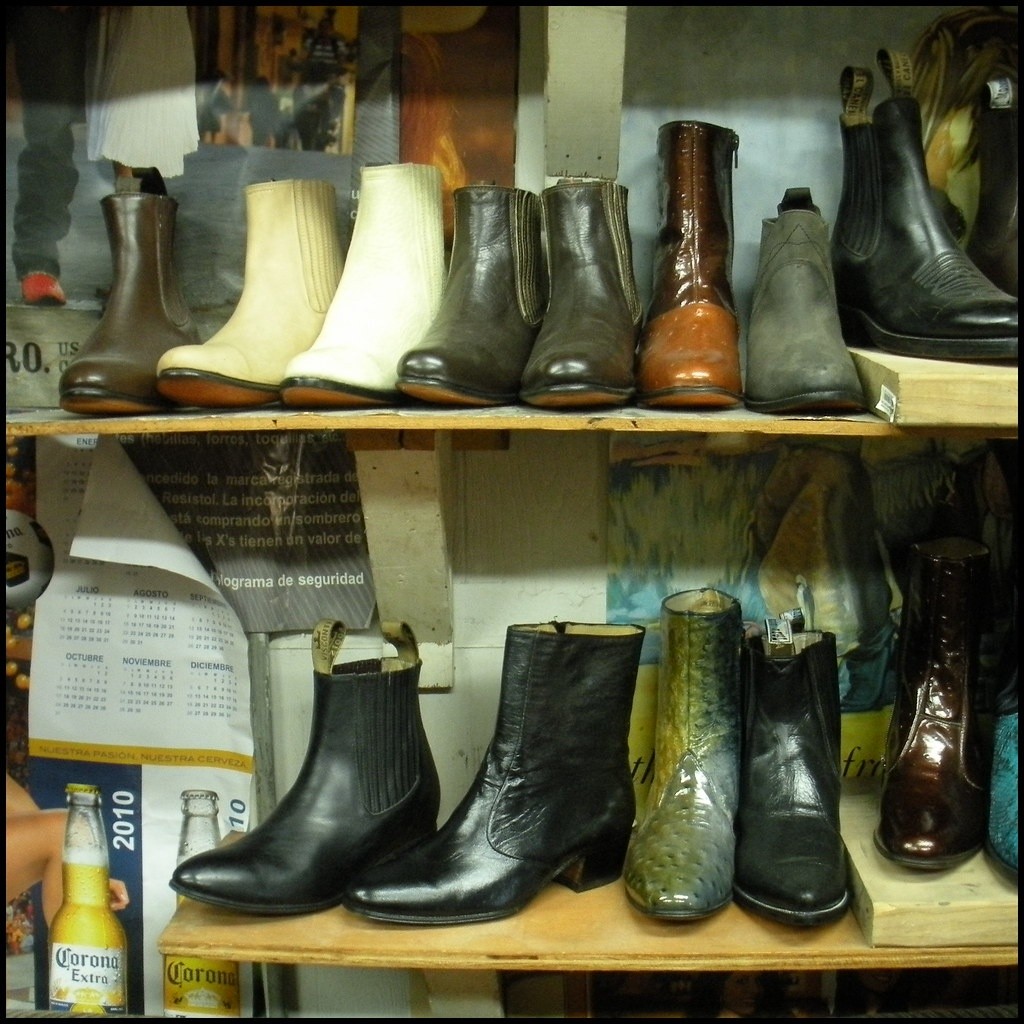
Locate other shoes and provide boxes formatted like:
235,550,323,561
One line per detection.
23,272,65,306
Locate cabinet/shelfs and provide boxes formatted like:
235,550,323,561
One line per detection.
6,343,1019,974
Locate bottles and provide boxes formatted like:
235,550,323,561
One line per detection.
47,783,240,1024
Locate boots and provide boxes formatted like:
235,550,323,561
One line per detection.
343,620,646,924
395,181,548,407
519,182,642,410
155,178,345,408
873,530,1003,871
278,163,447,411
169,617,440,917
827,48,1018,362
633,120,742,406
741,188,865,415
623,583,749,919
56,166,203,418
730,627,848,927
966,77,1018,298
